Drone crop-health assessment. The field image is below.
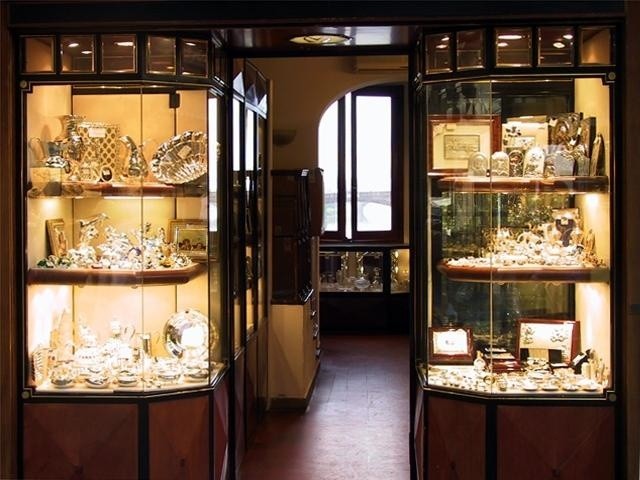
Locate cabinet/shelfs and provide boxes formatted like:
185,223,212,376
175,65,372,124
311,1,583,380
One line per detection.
16,29,272,480
271,168,324,409
320,241,411,335
411,15,629,480
